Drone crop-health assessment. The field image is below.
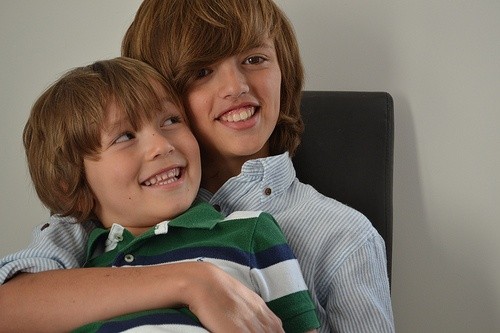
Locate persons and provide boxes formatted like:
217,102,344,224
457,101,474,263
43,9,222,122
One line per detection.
22,57,320,333
0,0,394,333
